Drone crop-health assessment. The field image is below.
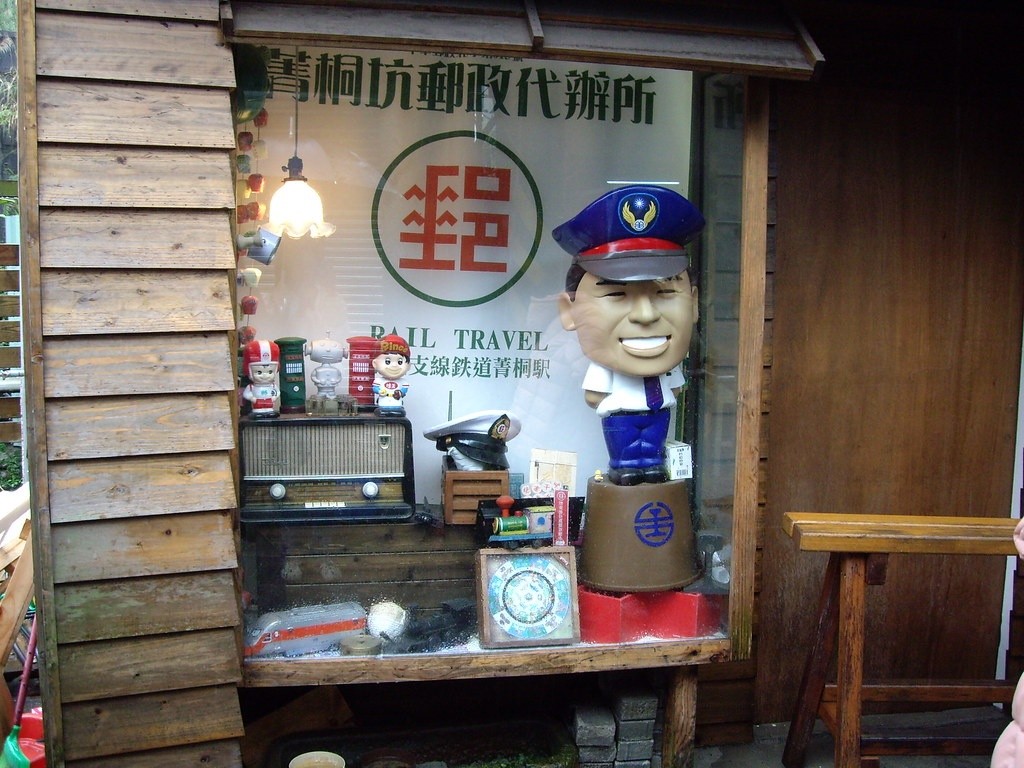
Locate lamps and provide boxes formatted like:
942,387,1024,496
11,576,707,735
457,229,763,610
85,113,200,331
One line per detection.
268,48,335,238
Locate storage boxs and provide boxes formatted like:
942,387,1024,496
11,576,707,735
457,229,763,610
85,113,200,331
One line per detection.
664,438,694,481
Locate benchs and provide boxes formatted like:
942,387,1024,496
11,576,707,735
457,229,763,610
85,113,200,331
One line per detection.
782,512,1024,767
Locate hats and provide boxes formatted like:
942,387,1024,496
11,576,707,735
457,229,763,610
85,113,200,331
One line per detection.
424,411,522,469
